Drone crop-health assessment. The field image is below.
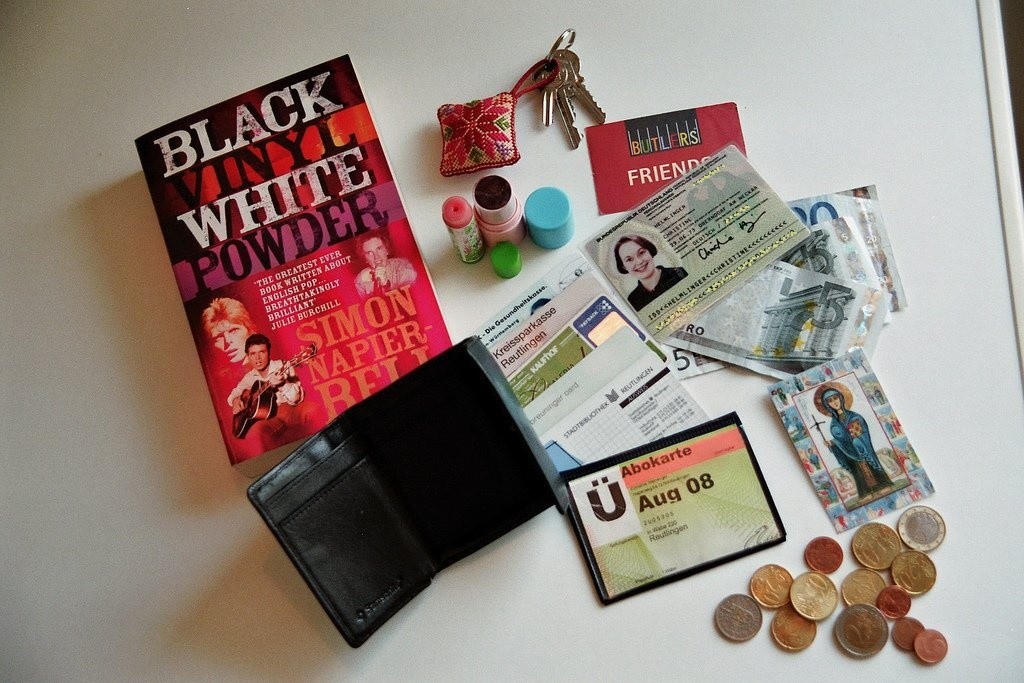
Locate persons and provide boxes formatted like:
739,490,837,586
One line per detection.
615,233,687,312
201,227,415,452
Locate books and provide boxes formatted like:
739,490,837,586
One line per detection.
132,54,449,469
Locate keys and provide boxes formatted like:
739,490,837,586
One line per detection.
533,29,606,151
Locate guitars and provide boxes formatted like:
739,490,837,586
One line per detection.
232,341,317,439
